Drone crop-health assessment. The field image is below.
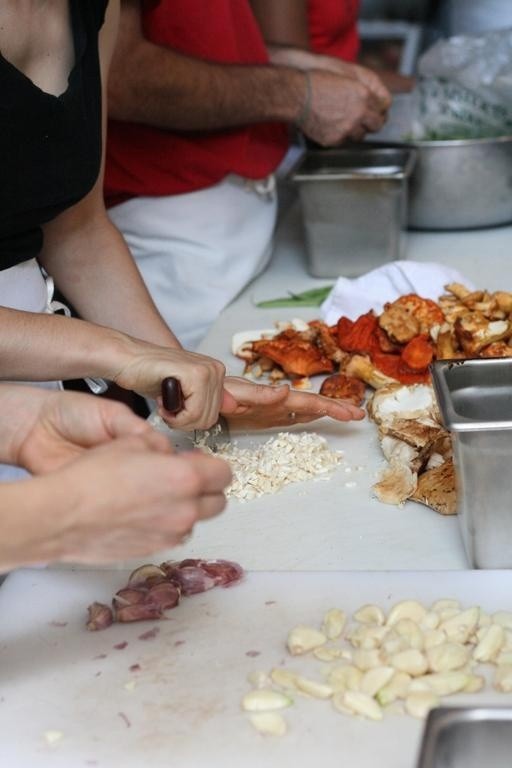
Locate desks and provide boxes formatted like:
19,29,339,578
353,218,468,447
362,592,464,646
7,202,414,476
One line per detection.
0,202,509,767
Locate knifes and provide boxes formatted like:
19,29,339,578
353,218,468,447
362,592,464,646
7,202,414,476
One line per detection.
162,374,231,452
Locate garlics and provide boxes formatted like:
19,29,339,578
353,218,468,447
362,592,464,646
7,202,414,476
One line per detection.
86,559,243,632
243,599,512,736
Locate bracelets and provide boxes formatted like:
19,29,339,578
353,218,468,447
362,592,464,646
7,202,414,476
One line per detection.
295,71,312,130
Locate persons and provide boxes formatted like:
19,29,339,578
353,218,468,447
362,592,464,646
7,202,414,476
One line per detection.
0,381,236,580
1,2,367,432
84,3,397,339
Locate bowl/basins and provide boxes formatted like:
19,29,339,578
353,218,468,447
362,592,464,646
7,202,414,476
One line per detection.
361,92,512,232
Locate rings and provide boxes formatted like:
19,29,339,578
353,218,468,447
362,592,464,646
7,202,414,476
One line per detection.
290,412,296,420
